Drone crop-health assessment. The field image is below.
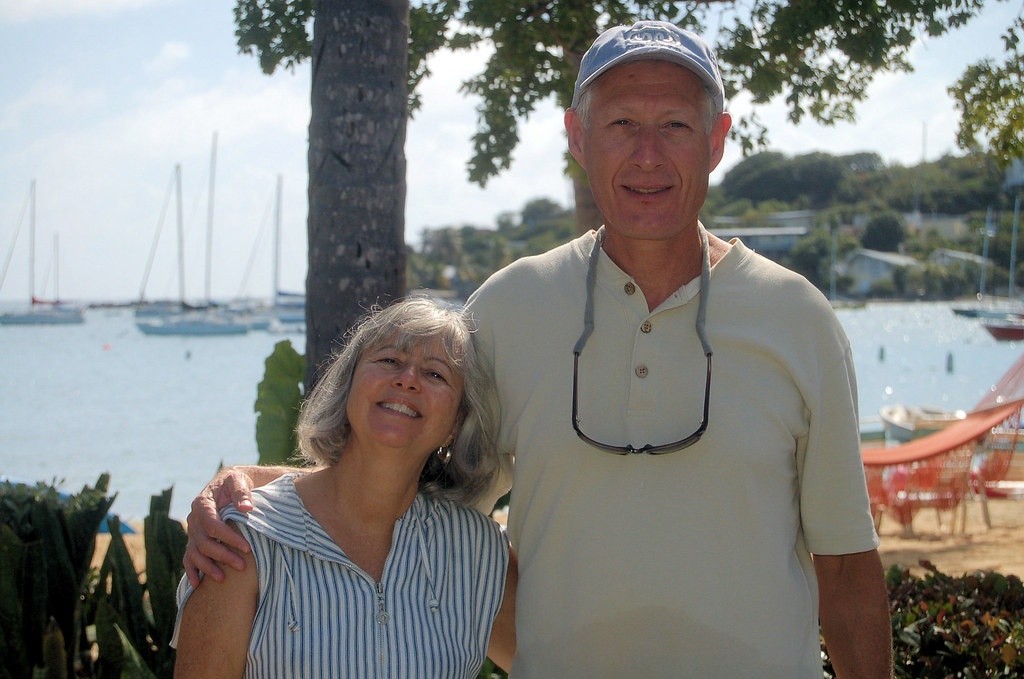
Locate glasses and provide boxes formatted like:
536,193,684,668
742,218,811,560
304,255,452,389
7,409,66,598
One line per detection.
572,353,713,456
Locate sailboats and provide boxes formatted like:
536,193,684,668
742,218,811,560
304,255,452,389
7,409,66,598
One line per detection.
0,176,84,326
951,197,1024,343
132,128,309,337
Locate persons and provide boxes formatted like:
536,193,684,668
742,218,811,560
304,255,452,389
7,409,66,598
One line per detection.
183,20,894,679
167,292,518,679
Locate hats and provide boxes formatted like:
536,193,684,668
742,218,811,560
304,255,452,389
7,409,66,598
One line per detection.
571,21,726,113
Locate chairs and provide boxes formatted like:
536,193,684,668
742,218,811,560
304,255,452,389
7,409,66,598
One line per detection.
861,353,1024,541
